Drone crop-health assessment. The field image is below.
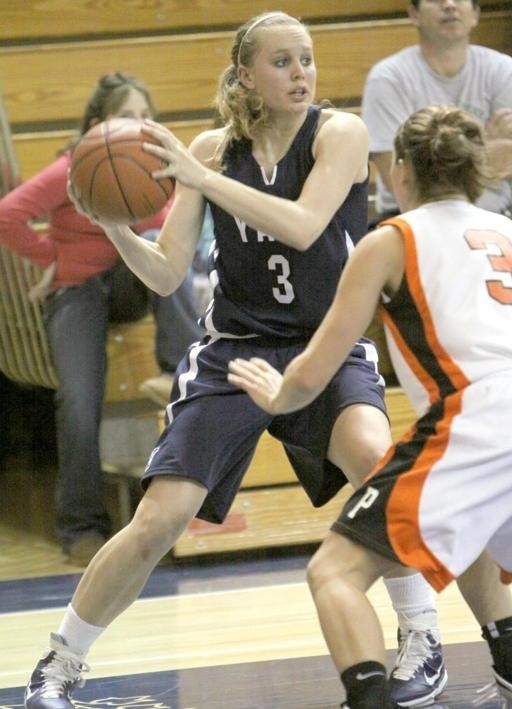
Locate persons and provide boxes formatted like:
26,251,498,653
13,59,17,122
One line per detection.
0,70,207,568
225,104,512,709
360,0,512,221
21,12,451,709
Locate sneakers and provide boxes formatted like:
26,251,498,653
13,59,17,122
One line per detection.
490,637,512,692
387,626,448,708
24,633,90,708
340,685,396,708
61,530,105,567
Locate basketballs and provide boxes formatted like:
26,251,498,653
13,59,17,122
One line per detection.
70,118,177,228
